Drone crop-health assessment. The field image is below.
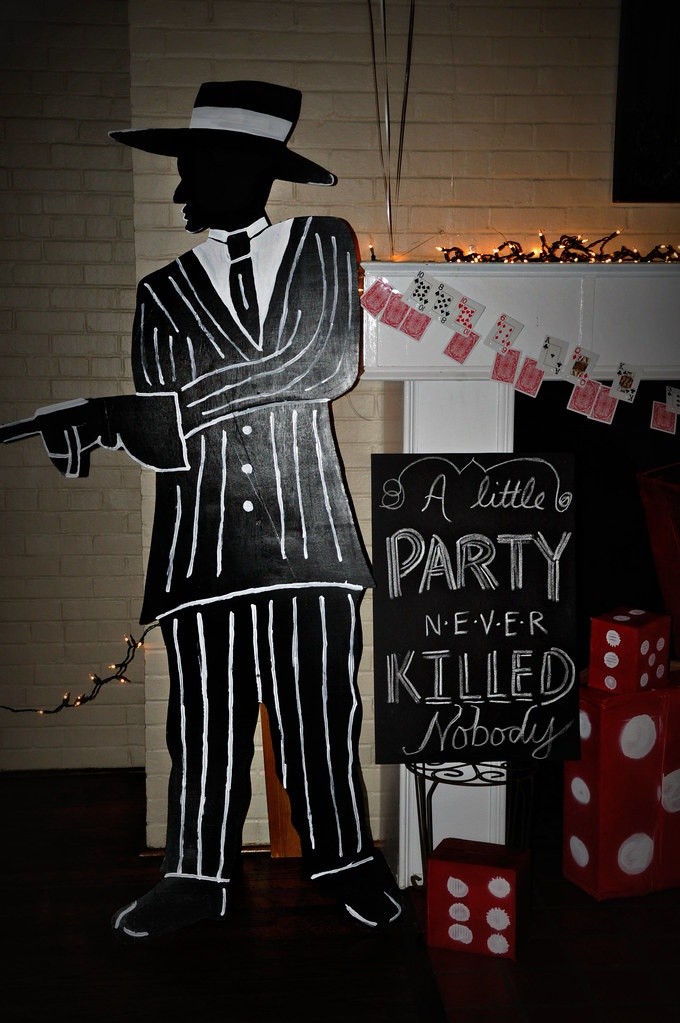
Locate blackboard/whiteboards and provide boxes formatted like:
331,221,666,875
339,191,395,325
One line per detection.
368,450,581,764
